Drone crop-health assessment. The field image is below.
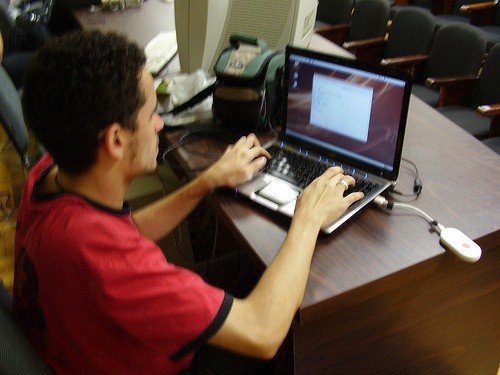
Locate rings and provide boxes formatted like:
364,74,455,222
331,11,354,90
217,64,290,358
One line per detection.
338,179,348,191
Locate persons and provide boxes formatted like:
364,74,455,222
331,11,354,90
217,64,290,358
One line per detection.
12,29,364,375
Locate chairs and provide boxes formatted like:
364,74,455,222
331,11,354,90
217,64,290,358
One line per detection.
0,65,46,170
315,0,499,153
1,272,59,375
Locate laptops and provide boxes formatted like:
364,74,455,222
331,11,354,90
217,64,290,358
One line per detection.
229,45,411,235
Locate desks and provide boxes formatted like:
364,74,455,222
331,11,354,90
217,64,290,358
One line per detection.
72,0,500,375
